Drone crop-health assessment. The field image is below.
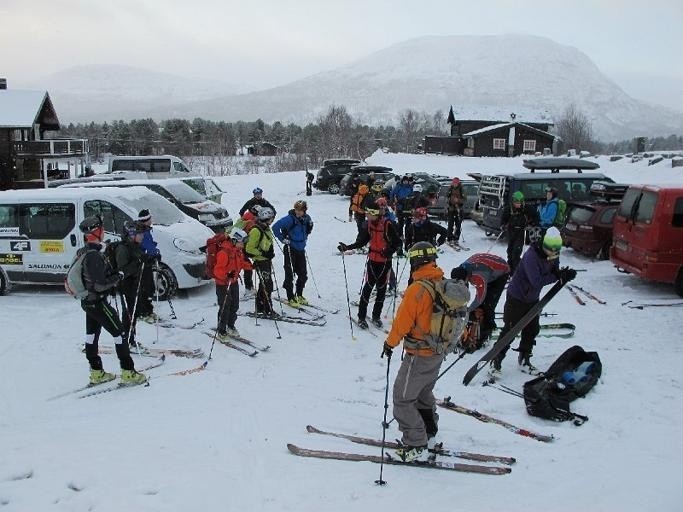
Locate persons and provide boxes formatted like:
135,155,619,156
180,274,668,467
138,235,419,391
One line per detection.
450,185,577,382
337,171,467,330
65,216,147,385
382,241,471,465
206,187,314,343
136,210,160,323
104,220,152,354
306,171,314,196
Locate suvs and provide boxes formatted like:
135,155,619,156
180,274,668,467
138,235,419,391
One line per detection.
376,168,485,221
311,157,365,197
555,180,634,260
470,153,619,242
336,164,398,196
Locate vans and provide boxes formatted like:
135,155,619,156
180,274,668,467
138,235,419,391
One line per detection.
38,150,237,239
608,183,683,300
0,185,222,298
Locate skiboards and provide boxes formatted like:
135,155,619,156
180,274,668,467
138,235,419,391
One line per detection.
287,427,516,475
83,343,204,359
565,283,606,304
445,239,469,251
437,399,553,442
73,355,207,397
153,315,204,329
491,324,575,338
347,313,389,336
201,327,271,356
275,294,340,317
352,289,403,306
463,279,568,386
247,311,325,325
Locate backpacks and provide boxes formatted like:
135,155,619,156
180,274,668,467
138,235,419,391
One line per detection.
64,250,107,300
414,278,470,356
206,233,230,278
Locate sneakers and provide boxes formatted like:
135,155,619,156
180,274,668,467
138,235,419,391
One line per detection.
481,367,502,386
288,295,309,308
357,318,368,329
89,369,116,387
118,368,147,384
215,325,242,345
519,358,540,376
371,317,383,328
253,309,281,320
138,313,160,324
385,434,437,465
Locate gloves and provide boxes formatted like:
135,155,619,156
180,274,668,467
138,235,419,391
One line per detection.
337,242,348,252
383,341,393,358
556,266,576,281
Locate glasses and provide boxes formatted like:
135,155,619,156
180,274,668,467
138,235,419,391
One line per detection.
545,186,553,193
362,206,379,216
90,215,104,230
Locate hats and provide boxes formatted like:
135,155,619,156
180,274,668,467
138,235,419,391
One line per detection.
543,227,562,251
513,192,523,203
451,267,467,281
80,218,104,233
137,209,151,221
452,178,459,184
549,187,559,199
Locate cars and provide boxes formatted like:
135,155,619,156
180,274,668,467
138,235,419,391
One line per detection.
356,173,395,185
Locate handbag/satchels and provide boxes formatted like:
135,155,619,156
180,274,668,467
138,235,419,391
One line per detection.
524,345,601,422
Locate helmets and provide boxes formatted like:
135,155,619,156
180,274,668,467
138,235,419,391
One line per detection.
253,188,262,194
230,226,249,244
121,221,152,245
414,207,427,219
294,200,307,211
251,204,275,222
408,241,437,269
413,183,422,192
